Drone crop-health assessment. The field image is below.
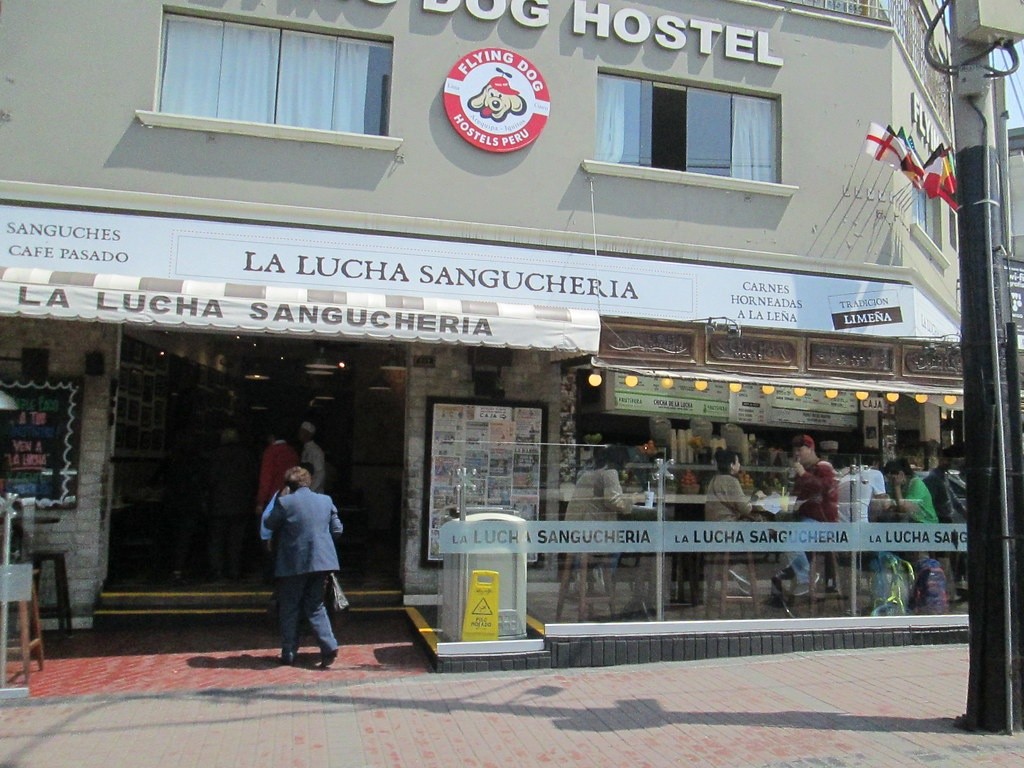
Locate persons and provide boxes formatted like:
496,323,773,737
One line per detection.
261,466,343,667
772,552,844,607
564,445,641,593
885,457,938,523
705,450,753,595
835,447,892,522
791,435,840,523
148,421,326,582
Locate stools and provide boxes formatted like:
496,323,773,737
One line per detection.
555,552,615,622
5,544,73,685
811,550,873,615
705,553,759,619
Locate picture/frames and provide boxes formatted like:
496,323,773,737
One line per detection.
117,338,238,452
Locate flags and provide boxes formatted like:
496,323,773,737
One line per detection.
885,125,925,191
862,120,907,173
924,145,958,199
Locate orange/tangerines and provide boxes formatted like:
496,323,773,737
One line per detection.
738,470,754,487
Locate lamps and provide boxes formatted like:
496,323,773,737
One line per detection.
587,368,928,404
895,333,962,355
688,317,742,338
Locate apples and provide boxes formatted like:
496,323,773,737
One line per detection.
680,468,697,484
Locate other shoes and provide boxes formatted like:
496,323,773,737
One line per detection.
792,573,819,596
319,649,338,667
277,652,293,663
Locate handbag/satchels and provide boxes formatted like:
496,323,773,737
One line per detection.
325,571,349,611
736,512,764,524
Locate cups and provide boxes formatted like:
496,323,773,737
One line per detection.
645,491,654,508
779,497,789,512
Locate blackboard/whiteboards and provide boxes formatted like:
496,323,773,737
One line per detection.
0,375,84,509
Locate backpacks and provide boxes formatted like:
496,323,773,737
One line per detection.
911,557,950,614
871,554,915,616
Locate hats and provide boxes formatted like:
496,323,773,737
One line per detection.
792,435,815,448
301,422,316,434
820,440,838,449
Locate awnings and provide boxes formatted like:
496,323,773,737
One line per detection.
0,264,601,355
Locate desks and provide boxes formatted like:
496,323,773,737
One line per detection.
1,516,60,638
